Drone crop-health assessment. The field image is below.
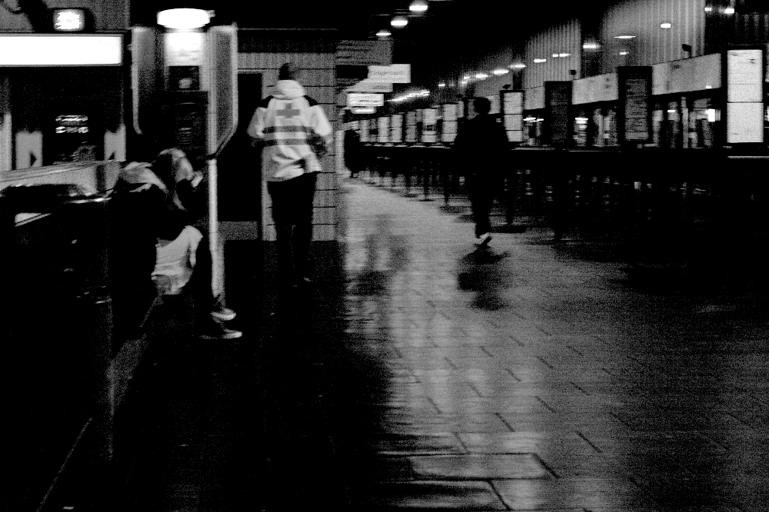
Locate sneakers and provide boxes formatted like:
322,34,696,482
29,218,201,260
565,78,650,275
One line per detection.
200,317,243,341
208,293,237,322
474,232,493,248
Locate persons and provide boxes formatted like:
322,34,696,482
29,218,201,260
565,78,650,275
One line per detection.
107,151,243,337
345,124,363,180
248,62,332,282
451,94,509,252
